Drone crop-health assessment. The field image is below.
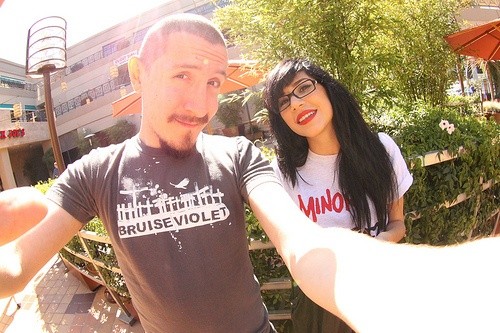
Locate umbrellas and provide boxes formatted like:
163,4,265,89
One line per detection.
110,57,266,119
443,17,500,63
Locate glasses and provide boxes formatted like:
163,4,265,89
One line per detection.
273,79,318,114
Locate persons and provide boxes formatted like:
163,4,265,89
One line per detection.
52,162,58,178
257,59,414,333
1,13,500,333
